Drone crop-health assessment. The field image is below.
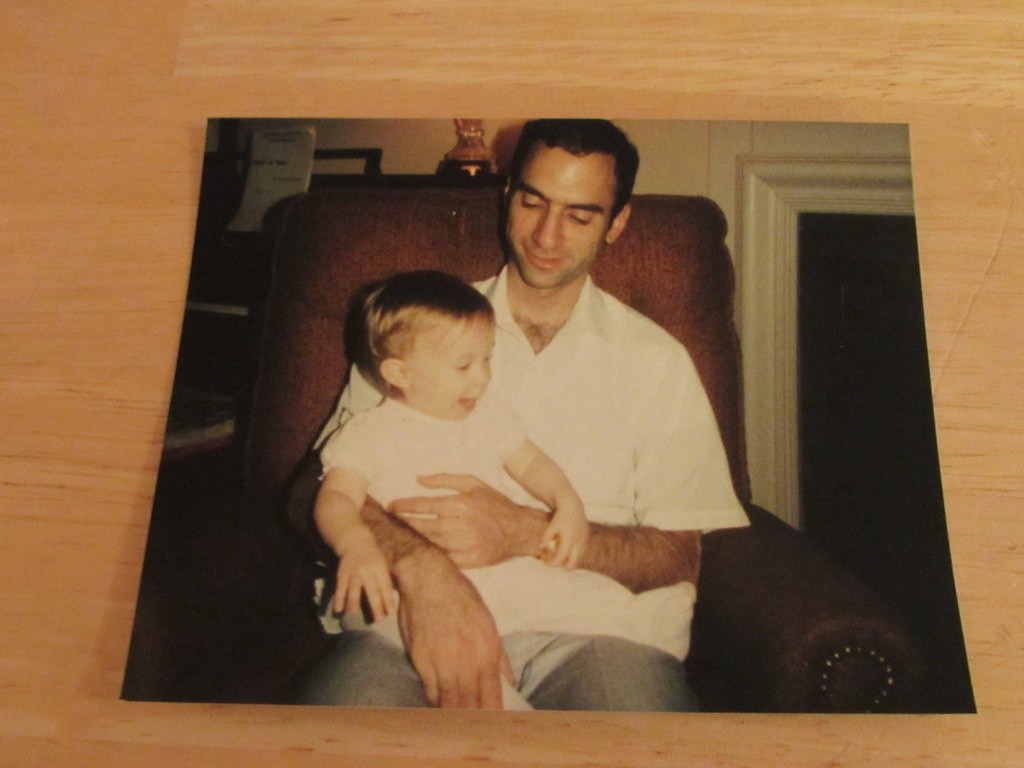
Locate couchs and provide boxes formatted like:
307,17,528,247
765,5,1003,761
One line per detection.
270,173,947,714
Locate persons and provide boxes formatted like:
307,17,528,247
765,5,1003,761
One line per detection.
286,120,750,711
